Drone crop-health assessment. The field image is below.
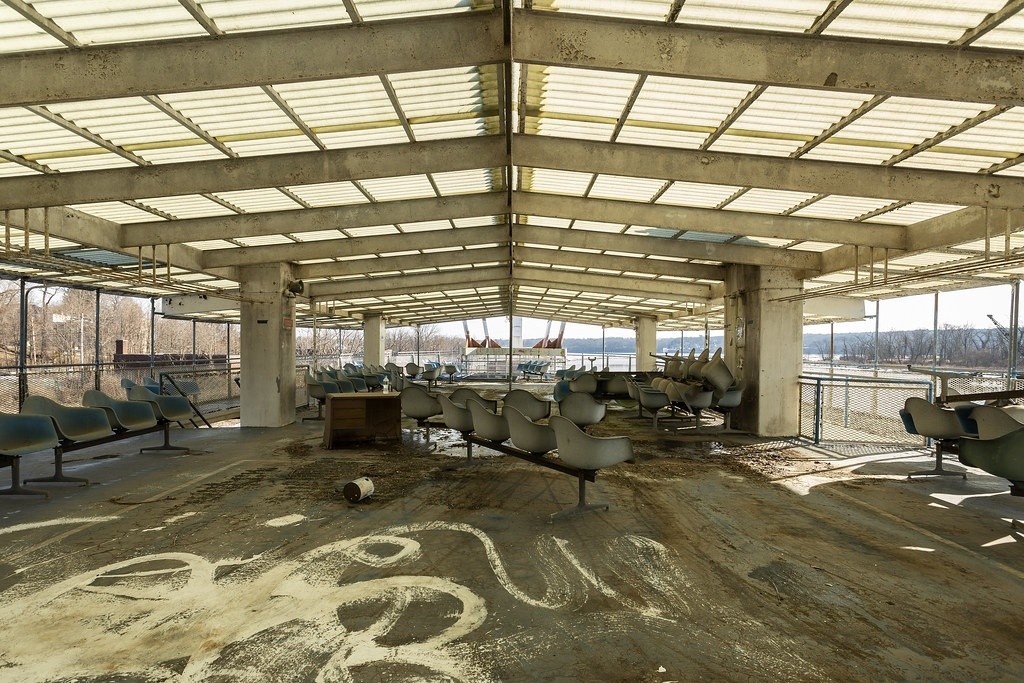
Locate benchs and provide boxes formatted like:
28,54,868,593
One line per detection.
899,385,1024,496
295,347,752,517
0,376,202,500
